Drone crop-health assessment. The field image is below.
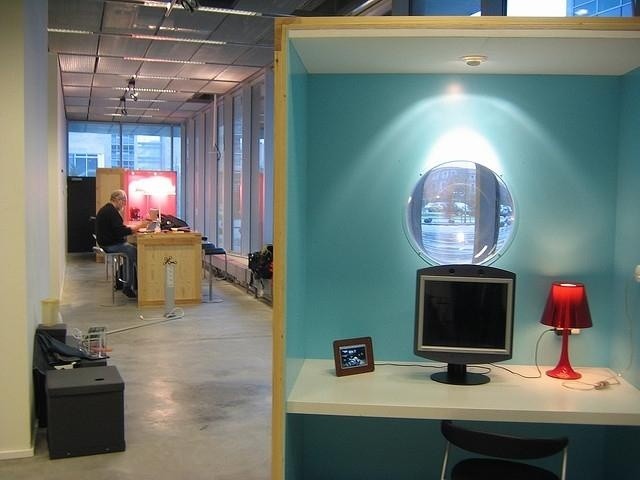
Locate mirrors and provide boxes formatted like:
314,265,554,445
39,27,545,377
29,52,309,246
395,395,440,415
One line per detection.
125,170,177,221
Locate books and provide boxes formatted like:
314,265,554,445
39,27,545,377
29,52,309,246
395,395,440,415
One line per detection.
170,226,190,232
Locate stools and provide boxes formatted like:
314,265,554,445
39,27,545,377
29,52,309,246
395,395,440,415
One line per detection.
201,237,228,303
89,217,130,307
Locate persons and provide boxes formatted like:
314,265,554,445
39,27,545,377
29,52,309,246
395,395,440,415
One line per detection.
95,190,150,298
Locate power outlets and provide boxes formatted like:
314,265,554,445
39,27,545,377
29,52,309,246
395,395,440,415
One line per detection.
556,326,580,335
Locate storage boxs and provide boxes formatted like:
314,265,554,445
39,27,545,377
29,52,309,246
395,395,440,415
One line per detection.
46,364,125,461
57,335,108,366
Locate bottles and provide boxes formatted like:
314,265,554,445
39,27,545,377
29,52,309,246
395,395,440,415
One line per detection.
155,217,161,233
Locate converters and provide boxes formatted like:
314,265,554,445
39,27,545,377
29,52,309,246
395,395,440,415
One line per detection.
554,327,573,336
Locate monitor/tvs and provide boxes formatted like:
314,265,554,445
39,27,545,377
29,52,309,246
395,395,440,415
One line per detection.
413,265,516,384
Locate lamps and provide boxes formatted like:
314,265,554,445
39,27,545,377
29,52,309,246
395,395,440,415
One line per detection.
120,78,139,116
135,188,152,213
136,187,176,220
542,279,592,379
177,1,198,14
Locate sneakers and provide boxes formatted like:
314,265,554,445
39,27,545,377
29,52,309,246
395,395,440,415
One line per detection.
122,287,136,299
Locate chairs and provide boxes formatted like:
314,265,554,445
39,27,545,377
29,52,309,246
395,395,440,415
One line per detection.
438,419,569,479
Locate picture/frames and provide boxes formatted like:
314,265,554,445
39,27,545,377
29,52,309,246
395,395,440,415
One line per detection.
332,335,375,378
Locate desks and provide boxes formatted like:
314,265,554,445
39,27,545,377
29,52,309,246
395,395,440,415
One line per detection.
126,232,202,305
287,357,640,426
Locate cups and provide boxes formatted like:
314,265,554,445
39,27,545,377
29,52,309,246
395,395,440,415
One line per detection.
40,297,59,327
